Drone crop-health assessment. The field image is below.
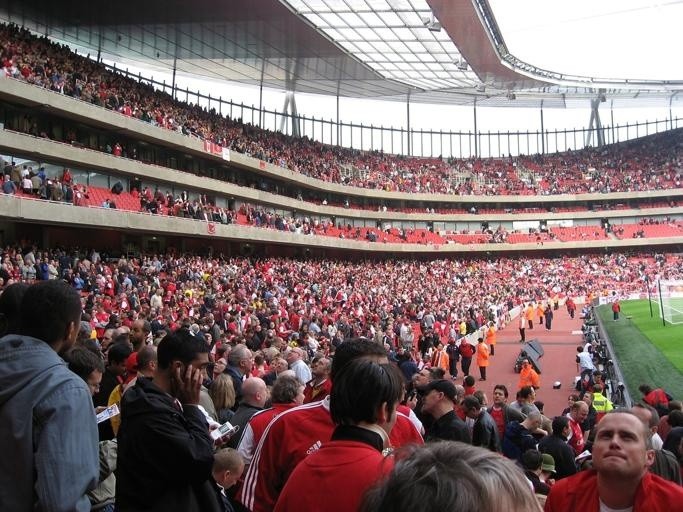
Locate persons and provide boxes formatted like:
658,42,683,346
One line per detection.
1,21,682,510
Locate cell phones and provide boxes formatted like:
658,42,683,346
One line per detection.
410,392,417,401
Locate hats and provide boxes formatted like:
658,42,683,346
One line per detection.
415,379,458,396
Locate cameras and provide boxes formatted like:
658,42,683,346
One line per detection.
210,421,238,441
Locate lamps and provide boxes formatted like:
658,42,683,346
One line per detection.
418,11,607,103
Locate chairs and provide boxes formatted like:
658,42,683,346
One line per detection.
447,252,681,301
0,123,682,245
